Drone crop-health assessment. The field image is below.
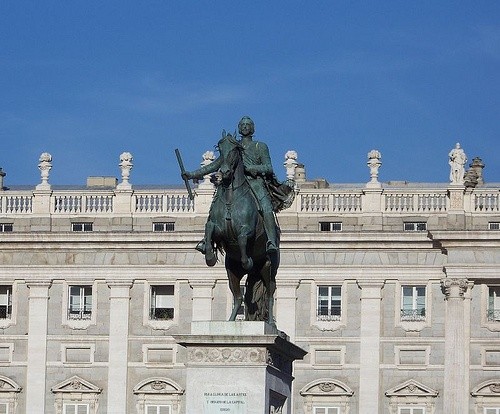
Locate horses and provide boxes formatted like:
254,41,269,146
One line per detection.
204,129,281,328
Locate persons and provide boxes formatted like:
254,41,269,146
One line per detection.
448,143,468,185
181,116,278,254
